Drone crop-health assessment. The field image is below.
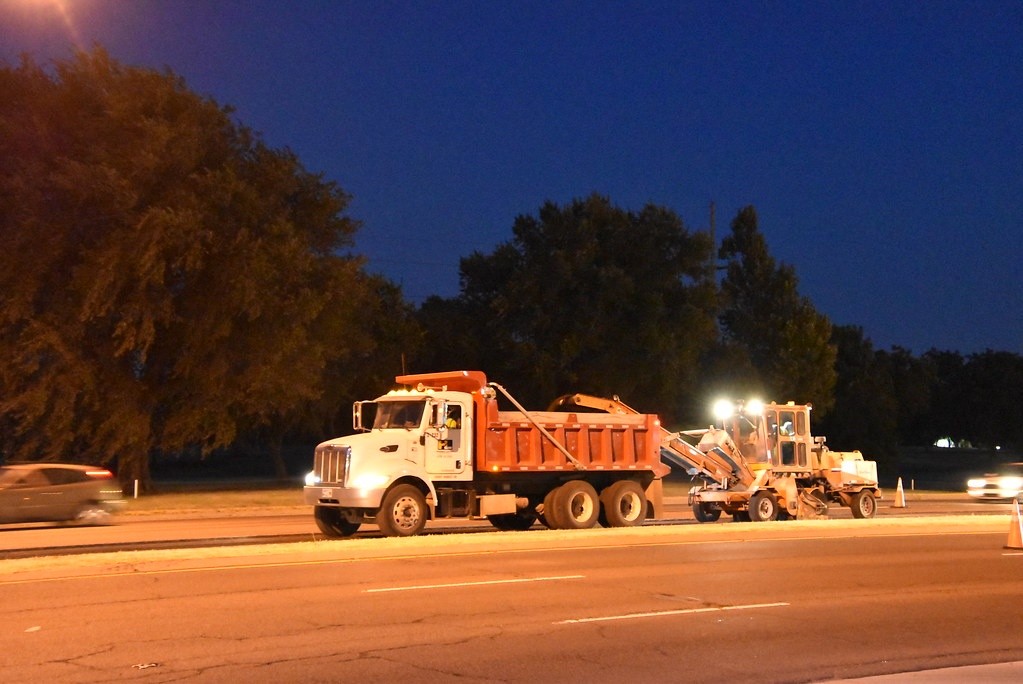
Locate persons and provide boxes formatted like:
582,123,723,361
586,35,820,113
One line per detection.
438,409,457,450
406,417,415,426
770,415,793,465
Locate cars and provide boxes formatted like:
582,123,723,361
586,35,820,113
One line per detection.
967,463,1023,504
0,464,127,526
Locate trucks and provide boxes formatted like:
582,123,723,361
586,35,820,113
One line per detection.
300,369,671,536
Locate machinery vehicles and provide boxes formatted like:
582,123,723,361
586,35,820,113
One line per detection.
557,392,883,523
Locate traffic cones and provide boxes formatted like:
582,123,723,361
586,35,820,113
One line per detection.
1002,499,1023,550
890,477,908,508
